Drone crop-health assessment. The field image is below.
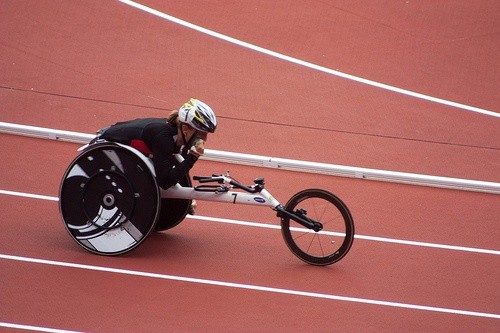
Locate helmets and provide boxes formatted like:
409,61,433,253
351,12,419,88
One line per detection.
177,97,217,133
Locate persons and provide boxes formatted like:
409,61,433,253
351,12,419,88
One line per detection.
82,98,217,215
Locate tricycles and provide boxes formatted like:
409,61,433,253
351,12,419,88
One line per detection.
58,143,354,266
